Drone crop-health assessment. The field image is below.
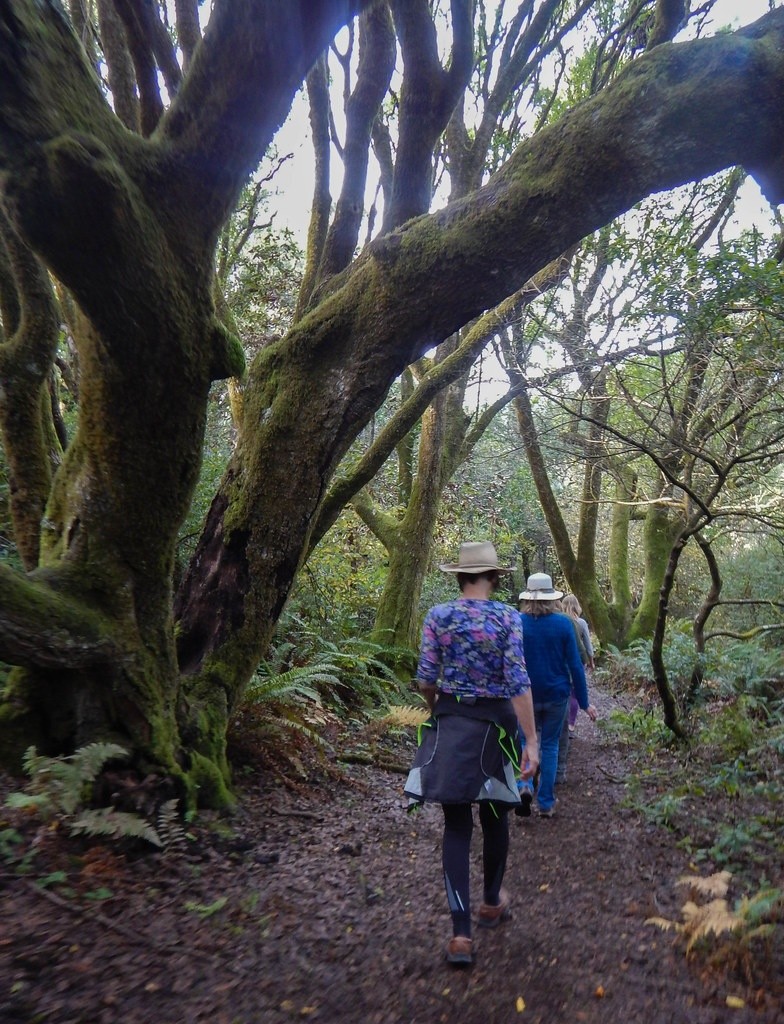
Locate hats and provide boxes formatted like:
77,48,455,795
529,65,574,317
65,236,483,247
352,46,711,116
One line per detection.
519,573,563,601
439,540,518,575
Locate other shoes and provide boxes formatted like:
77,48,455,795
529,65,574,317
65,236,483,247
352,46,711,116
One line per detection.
567,730,575,738
444,937,472,962
538,808,556,818
555,774,568,784
515,787,532,818
478,888,509,927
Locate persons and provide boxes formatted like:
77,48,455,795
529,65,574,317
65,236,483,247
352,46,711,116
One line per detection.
515,571,597,819
403,539,540,968
555,593,595,784
